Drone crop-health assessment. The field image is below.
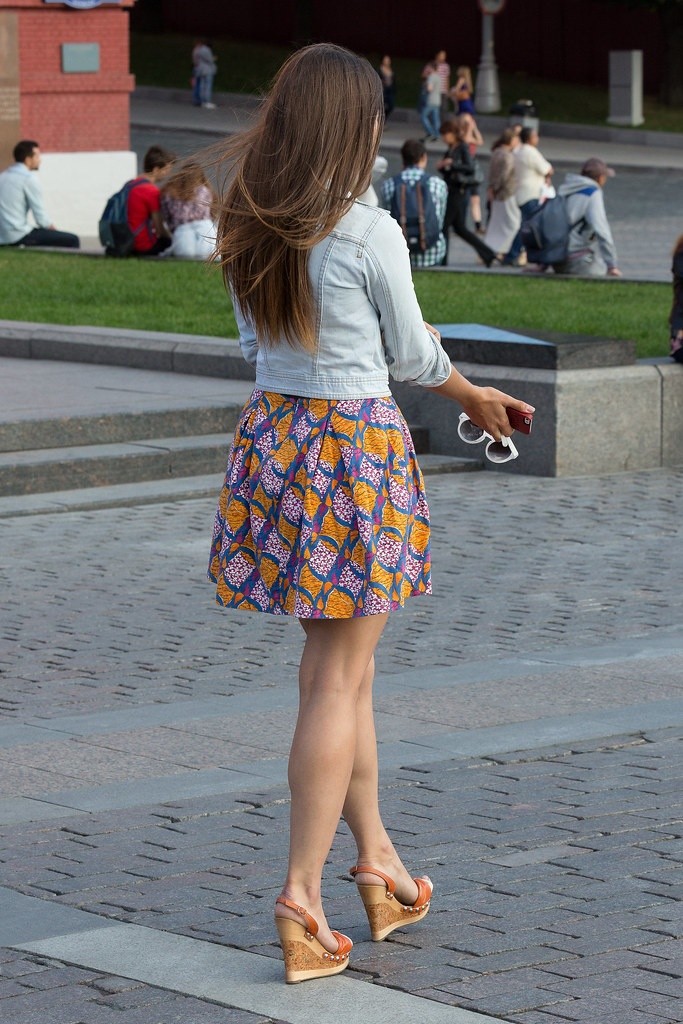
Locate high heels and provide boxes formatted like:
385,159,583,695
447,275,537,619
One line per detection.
349,865,434,942
273,896,354,983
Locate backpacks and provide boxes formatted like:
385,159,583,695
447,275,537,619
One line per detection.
392,171,440,254
520,186,598,263
98,179,153,252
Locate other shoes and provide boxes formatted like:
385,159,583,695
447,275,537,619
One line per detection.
501,258,527,268
204,102,216,110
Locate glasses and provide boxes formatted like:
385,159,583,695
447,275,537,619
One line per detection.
456,412,519,464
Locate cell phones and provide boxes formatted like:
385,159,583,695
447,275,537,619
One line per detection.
505,407,533,434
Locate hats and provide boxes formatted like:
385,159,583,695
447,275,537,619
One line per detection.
582,158,615,178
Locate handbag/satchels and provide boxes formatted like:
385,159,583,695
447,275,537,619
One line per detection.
440,92,456,114
455,162,486,197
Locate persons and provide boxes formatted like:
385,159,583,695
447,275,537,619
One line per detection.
102,148,220,261
358,112,622,276
376,55,395,124
191,34,217,109
418,47,477,142
207,44,535,983
0,139,80,248
669,234,683,365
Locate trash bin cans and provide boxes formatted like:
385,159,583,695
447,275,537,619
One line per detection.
508,99,540,134
